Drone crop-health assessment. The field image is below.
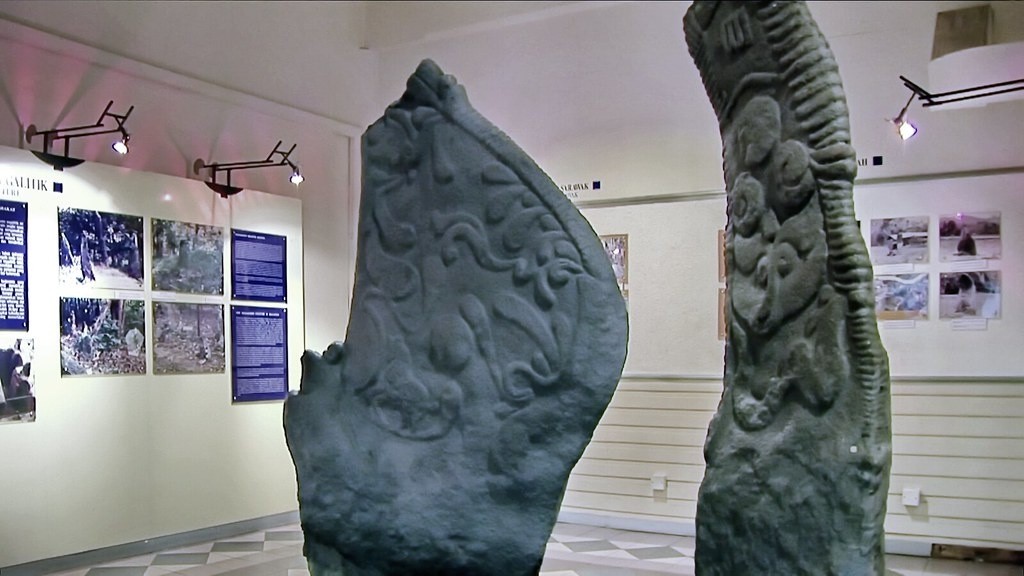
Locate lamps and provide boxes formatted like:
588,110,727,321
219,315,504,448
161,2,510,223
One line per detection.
29,99,134,171
894,91,918,140
203,140,305,198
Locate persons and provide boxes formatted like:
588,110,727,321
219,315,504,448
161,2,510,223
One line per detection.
956,226,976,257
